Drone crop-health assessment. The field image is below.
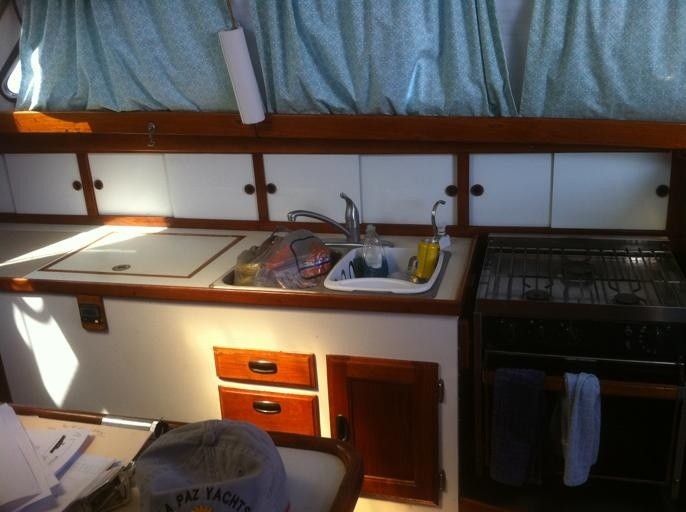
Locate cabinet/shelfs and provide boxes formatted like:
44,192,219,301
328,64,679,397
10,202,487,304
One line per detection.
1,150,673,235
2,294,458,512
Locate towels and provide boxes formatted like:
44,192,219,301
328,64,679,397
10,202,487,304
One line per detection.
561,372,602,487
492,367,546,489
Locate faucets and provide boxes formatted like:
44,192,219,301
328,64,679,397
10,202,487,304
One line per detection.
285,190,358,243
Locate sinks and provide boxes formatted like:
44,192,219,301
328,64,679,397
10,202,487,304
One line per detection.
207,239,450,306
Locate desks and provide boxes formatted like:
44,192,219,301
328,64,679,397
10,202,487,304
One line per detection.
0,403,363,512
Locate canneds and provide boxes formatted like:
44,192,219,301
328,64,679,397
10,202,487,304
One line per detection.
414,237,441,281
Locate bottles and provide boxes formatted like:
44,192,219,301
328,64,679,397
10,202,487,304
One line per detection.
362,224,383,269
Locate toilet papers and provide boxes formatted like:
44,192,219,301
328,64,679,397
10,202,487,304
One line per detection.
218,26,264,125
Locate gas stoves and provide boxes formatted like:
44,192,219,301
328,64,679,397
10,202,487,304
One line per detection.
474,232,685,384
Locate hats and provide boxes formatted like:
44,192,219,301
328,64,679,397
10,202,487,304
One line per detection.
136,419,289,512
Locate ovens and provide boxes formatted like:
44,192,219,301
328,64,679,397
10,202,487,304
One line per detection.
471,350,686,512
